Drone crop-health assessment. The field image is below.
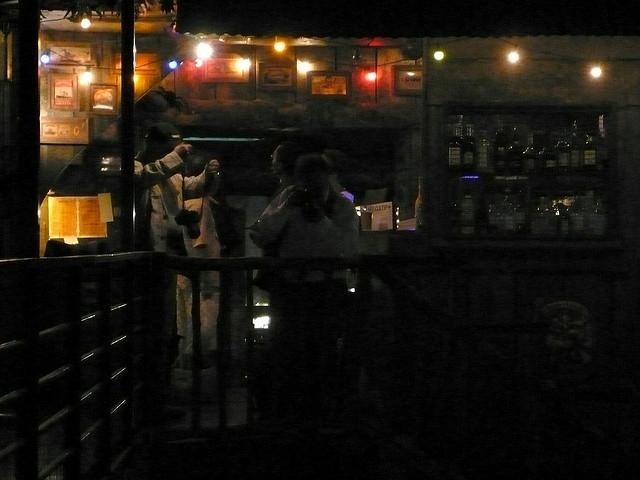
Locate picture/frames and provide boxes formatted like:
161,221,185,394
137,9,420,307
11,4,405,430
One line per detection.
393,64,424,96
90,83,118,116
200,52,352,105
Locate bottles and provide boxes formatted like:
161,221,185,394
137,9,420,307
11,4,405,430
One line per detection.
457,175,608,239
448,112,599,172
414,175,429,234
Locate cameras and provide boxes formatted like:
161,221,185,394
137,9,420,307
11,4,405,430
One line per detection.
175,208,201,239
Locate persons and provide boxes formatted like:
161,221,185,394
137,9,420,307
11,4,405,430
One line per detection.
249,153,360,433
130,122,224,436
169,149,224,371
252,139,306,319
